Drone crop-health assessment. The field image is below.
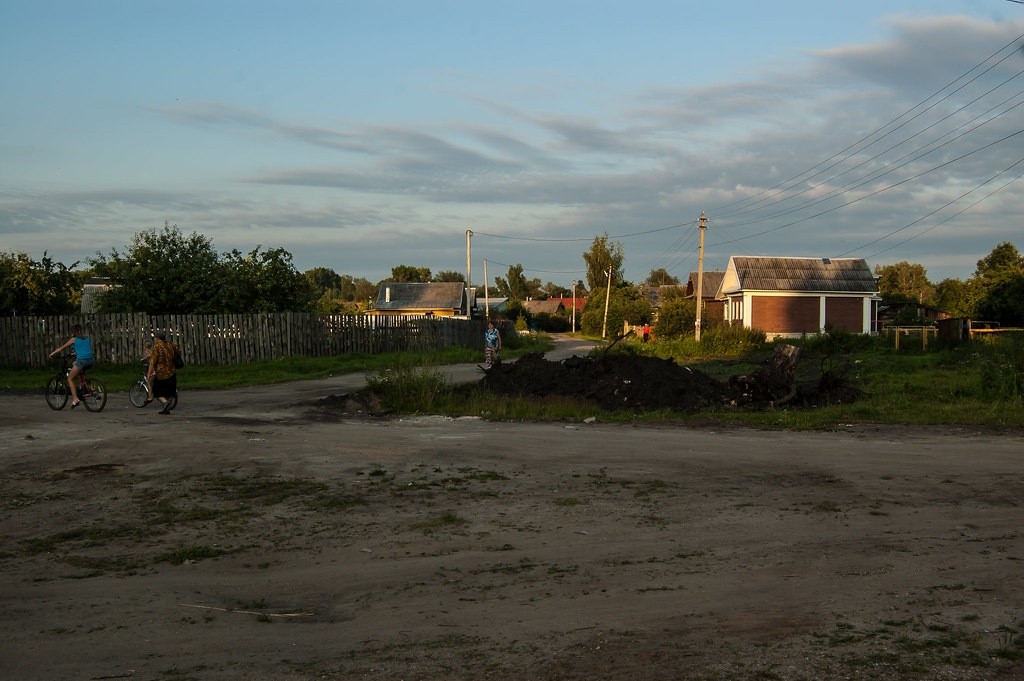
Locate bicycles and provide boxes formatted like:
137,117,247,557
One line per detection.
45,356,108,412
128,359,179,410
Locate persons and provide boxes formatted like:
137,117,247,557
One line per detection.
49,324,95,410
643,321,650,343
483,321,502,369
143,330,177,415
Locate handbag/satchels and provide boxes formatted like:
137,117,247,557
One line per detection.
174,352,184,370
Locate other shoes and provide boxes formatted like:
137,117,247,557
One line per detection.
163,401,172,411
158,411,170,414
70,399,79,409
144,399,153,403
